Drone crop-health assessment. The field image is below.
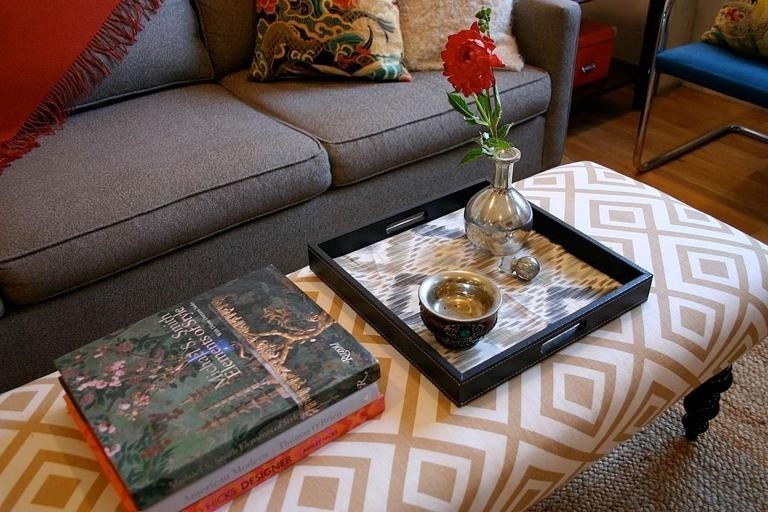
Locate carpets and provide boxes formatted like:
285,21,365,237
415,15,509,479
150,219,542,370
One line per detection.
521,331,768,511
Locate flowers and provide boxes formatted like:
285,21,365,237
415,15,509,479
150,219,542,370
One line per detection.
440,8,513,156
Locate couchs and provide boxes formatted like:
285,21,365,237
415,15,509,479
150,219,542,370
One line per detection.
0,0,585,512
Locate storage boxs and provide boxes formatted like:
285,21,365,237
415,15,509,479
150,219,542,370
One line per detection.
573,26,615,86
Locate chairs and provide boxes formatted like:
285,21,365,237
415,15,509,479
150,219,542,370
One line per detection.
632,0,768,175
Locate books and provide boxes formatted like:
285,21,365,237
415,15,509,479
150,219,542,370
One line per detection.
55,264,385,510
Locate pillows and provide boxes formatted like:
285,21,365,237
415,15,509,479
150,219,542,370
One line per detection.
703,0,767,65
394,0,525,73
248,0,411,84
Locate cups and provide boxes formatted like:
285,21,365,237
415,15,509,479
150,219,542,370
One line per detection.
418,270,503,351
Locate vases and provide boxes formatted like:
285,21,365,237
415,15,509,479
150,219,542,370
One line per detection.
465,145,533,254
